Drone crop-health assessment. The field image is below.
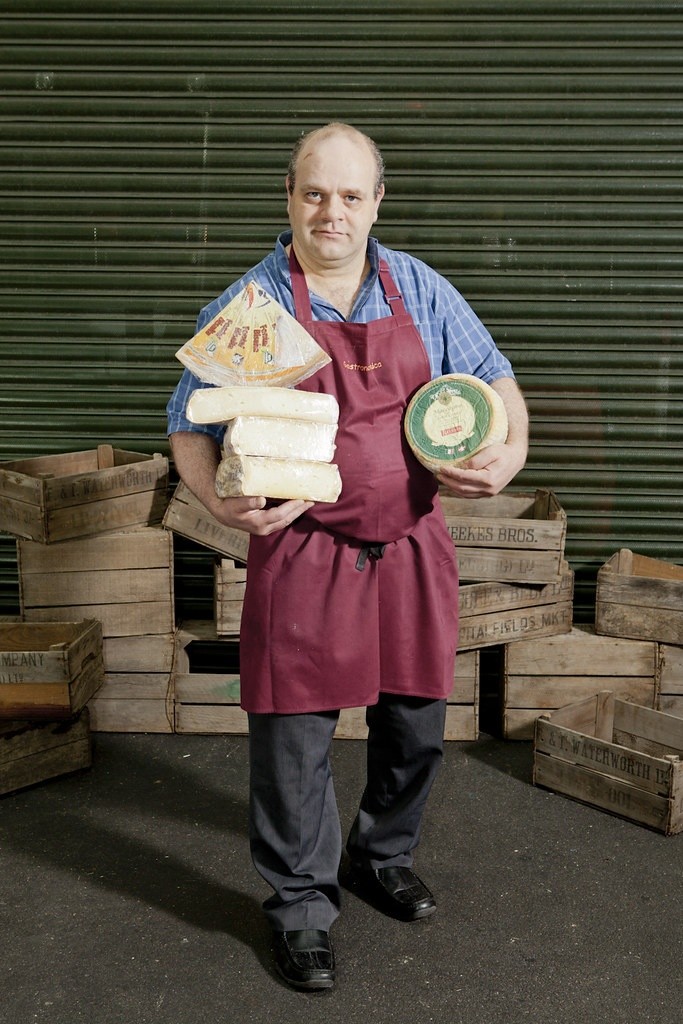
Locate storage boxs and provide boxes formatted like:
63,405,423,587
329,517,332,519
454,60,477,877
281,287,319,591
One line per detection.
0,442,681,835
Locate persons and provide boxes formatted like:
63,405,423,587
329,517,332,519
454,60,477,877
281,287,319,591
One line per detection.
165,121,531,990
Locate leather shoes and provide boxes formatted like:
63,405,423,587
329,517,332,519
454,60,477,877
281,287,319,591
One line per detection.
274,929,339,989
360,868,438,920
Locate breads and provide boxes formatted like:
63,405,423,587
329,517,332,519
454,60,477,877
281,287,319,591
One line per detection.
175,278,509,505
